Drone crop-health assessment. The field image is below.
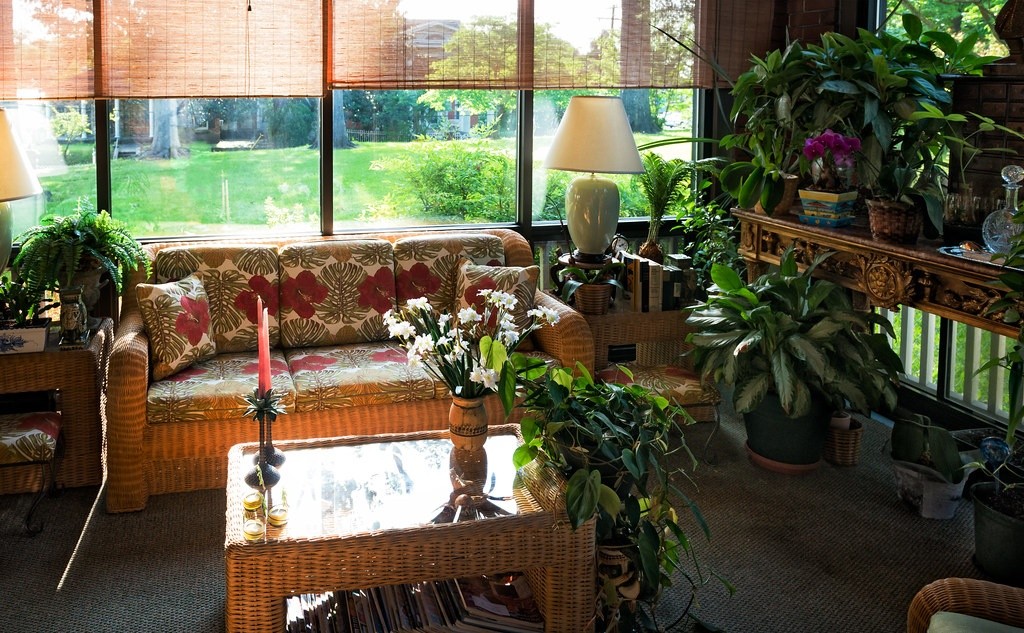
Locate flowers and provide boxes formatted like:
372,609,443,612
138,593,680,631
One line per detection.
383,287,560,399
803,127,863,191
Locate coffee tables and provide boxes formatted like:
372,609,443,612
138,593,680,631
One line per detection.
223,421,598,633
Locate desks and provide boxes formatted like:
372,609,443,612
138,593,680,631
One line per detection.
731,190,1024,341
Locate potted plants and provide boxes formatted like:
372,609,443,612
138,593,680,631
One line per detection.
559,150,745,315
681,242,1023,474
636,12,1005,219
834,92,953,245
0,195,155,355
480,334,737,633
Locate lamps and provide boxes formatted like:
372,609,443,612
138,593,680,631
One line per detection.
1,106,69,248
242,295,286,490
542,94,645,264
0,104,44,279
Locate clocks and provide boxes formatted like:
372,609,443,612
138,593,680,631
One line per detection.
610,234,629,255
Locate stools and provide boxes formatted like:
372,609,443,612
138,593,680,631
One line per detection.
596,362,723,469
1,411,73,537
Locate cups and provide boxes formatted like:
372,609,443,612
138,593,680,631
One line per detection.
244,485,289,540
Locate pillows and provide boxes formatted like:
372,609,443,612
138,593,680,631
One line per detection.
451,253,540,353
134,267,220,382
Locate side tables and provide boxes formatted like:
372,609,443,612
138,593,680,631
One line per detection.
544,283,707,374
1,317,114,490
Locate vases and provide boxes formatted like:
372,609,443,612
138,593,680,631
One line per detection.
242,464,291,542
884,422,1024,586
449,390,489,451
798,181,859,228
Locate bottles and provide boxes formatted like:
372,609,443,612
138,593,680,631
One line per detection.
982,164,1024,258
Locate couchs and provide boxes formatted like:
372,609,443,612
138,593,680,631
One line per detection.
107,228,595,514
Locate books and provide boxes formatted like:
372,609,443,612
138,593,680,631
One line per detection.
283,570,544,632
614,251,686,313
